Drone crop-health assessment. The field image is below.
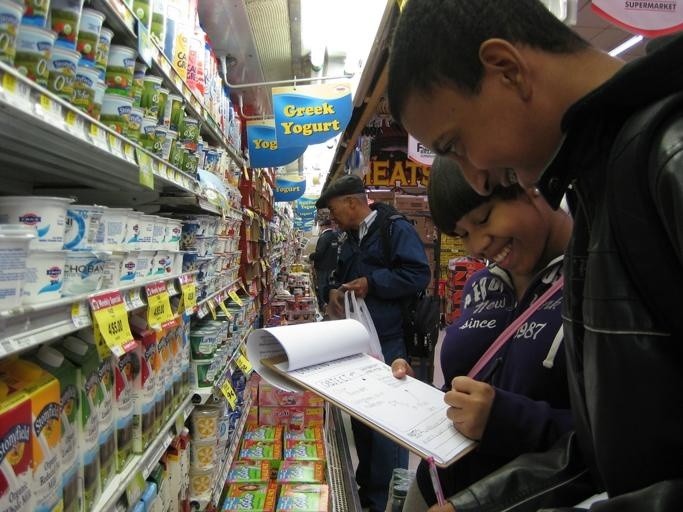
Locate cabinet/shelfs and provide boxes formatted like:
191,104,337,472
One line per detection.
2,12,358,494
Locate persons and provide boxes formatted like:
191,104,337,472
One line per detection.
390,152,575,510
307,174,432,512
387,0,683,511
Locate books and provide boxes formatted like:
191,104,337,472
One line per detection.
243,320,482,468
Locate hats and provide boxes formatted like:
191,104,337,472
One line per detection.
315,175,365,209
319,220,331,226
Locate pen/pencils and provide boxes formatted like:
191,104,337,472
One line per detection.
426,456,446,508
481,357,503,383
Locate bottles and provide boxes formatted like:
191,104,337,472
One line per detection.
390,468,413,512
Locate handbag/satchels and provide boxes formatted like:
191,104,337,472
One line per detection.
411,297,440,350
